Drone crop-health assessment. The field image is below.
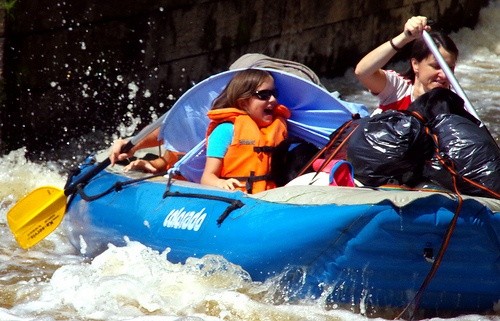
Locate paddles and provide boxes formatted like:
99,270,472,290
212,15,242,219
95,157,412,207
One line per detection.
6,110,171,251
407,20,500,154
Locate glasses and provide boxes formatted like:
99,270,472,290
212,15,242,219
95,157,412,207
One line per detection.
252,87,278,101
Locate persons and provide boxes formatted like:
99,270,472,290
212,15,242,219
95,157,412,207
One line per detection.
109,68,290,195
355,15,459,111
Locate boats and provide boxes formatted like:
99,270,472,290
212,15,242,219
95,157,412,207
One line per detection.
60,52,500,313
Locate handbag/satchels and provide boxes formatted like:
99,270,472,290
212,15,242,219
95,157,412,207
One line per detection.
269,136,324,187
